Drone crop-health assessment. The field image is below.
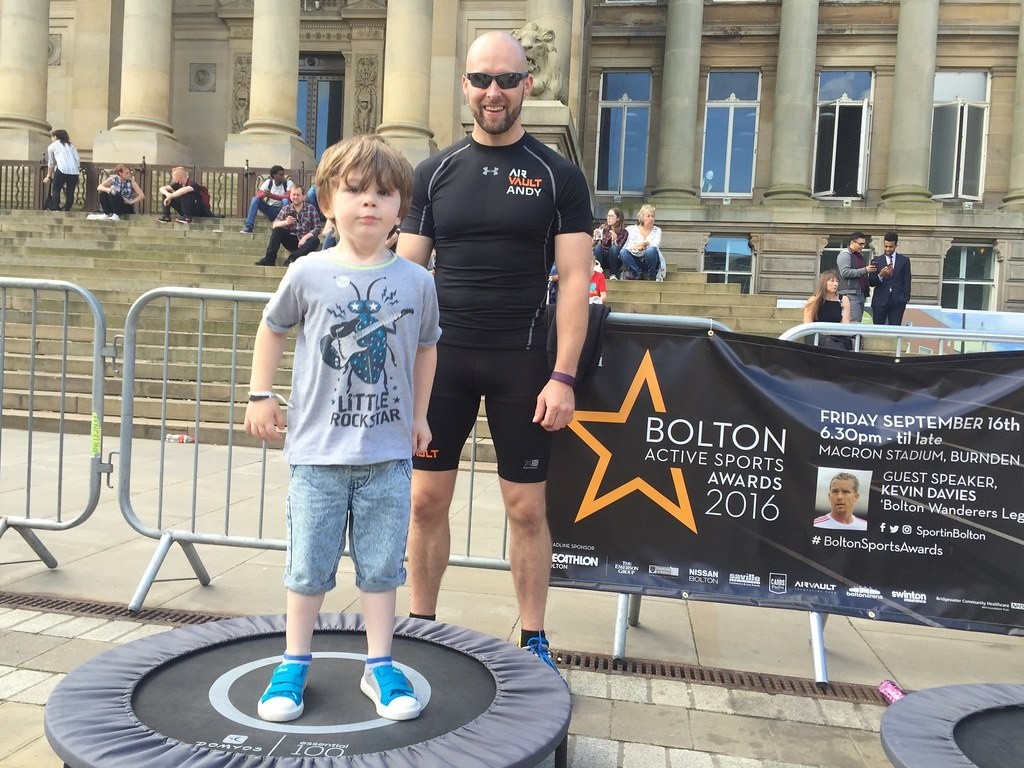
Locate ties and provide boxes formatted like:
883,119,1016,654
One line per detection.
889,256,894,277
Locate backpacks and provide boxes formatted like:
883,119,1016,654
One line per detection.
199,185,210,208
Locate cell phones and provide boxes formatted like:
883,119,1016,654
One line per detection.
872,261,878,267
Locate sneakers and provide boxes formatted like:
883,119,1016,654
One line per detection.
360,664,421,721
517,629,569,688
257,663,310,722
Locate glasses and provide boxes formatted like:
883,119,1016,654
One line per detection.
606,215,617,217
853,240,867,246
463,72,528,90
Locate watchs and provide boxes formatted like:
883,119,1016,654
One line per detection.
46,175,50,178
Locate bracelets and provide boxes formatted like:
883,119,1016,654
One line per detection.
247,391,294,410
549,371,576,386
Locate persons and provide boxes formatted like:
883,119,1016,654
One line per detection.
593,207,628,281
42,130,81,211
97,164,146,221
804,270,853,350
306,185,400,253
815,472,869,533
240,165,294,234
157,166,200,224
618,204,666,281
548,256,607,305
835,231,877,323
869,232,912,326
396,29,595,670
241,131,443,724
254,184,321,267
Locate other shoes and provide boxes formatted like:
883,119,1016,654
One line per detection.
240,228,254,234
636,274,643,280
647,274,656,281
610,274,617,280
255,258,276,266
284,254,298,267
157,217,172,223
59,206,70,211
108,213,120,221
95,213,108,220
52,206,60,211
175,216,192,223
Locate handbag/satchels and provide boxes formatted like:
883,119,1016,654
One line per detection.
43,177,52,210
